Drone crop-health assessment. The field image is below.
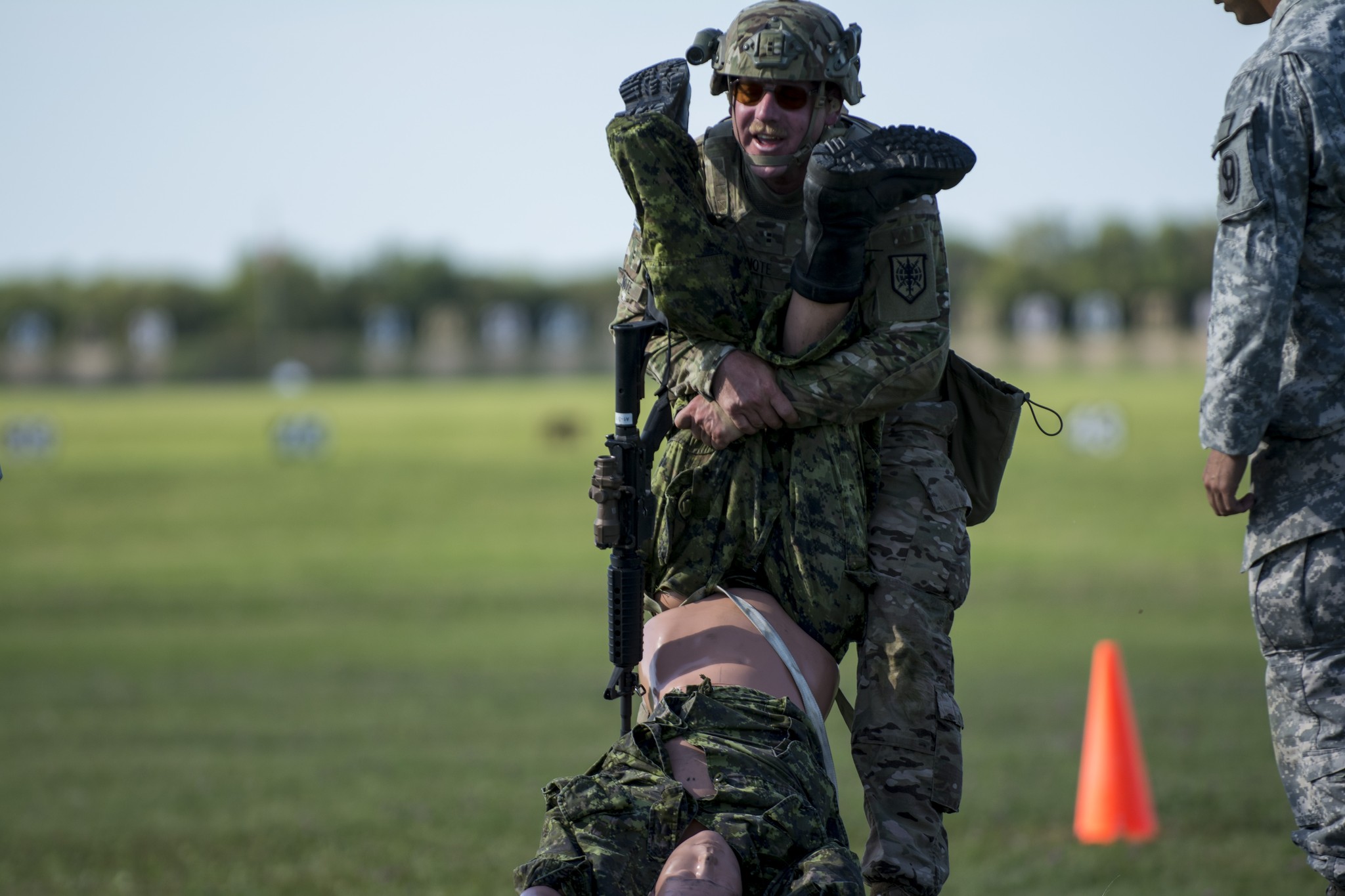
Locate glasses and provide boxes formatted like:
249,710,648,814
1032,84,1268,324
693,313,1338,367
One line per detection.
729,78,819,112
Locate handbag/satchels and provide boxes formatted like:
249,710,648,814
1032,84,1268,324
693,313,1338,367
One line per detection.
935,349,1064,527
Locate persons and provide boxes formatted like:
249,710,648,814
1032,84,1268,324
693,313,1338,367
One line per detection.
512,56,974,894
608,0,970,896
1194,0,1342,895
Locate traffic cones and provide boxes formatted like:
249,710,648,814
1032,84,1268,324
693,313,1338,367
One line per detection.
1072,643,1160,840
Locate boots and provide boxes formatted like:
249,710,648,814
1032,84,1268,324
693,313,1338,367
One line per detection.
615,58,693,133
790,123,977,304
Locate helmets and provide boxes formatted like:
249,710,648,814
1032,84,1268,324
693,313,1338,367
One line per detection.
710,0,865,106
